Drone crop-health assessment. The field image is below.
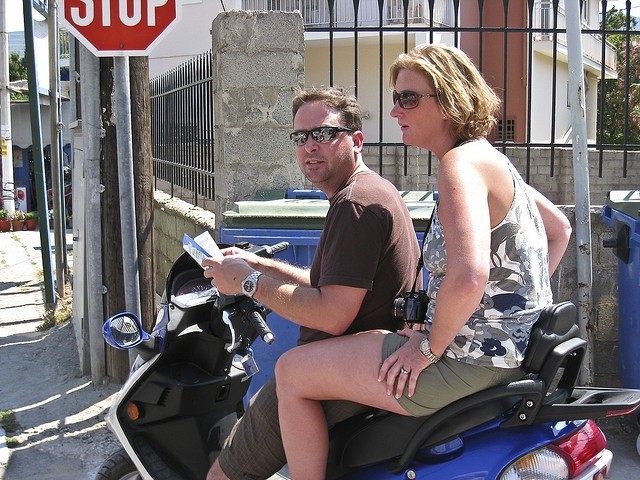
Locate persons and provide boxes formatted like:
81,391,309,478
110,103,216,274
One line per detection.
271,42,577,480
202,82,428,479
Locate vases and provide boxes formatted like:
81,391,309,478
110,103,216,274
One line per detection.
11,220,25,231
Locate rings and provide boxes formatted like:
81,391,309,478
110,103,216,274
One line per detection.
399,364,408,377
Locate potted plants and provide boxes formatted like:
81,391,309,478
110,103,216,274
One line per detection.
48,208,54,230
0,210,11,232
25,212,37,230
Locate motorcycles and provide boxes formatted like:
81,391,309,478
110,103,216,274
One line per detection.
93,238,640,480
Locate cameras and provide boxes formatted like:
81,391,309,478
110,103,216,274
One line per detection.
391,290,428,324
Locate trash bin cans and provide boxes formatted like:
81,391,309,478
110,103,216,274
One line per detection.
219,189,464,412
602,190,640,390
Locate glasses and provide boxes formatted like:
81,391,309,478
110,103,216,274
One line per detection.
392,90,437,108
290,127,354,146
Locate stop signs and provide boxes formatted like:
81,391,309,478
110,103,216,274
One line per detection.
59,0,179,57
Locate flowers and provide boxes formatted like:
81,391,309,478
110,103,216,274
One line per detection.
7,210,25,221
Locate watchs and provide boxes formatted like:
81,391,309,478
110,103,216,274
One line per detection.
415,333,437,366
239,270,266,299
